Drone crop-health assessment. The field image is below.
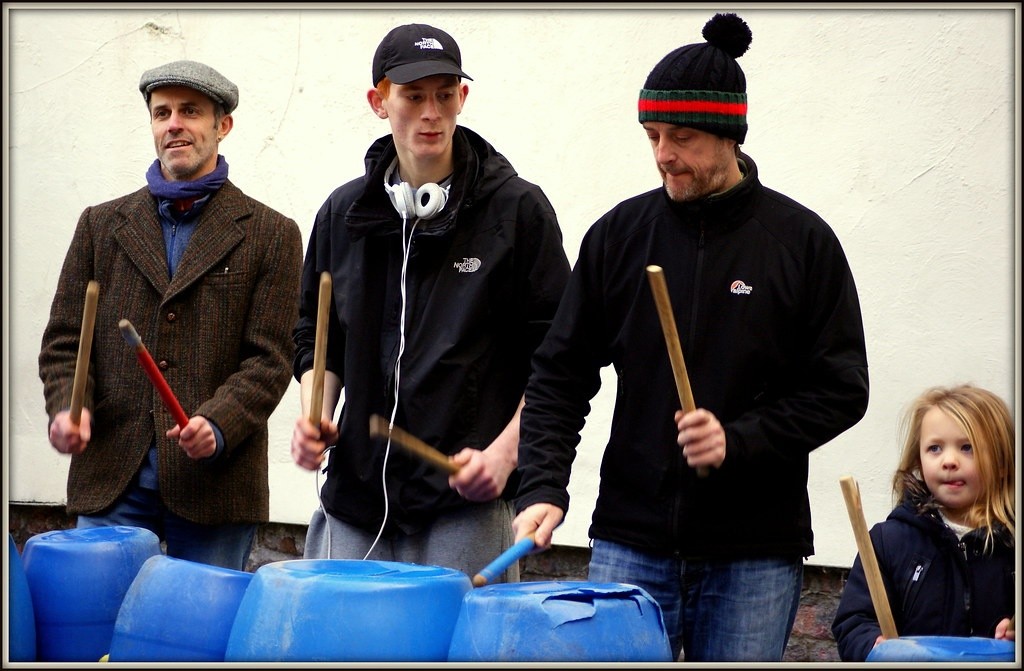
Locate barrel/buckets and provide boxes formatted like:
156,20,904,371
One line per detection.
9,534,37,663
866,636,1015,662
21,526,159,663
449,581,674,662
225,558,473,662
108,556,254,662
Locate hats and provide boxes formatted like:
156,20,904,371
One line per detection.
372,24,474,89
140,61,239,115
637,12,753,144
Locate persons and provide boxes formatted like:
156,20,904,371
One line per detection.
293,24,573,584
512,14,869,662
40,60,304,573
831,382,1015,662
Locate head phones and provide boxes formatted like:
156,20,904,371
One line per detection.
383,155,452,220
